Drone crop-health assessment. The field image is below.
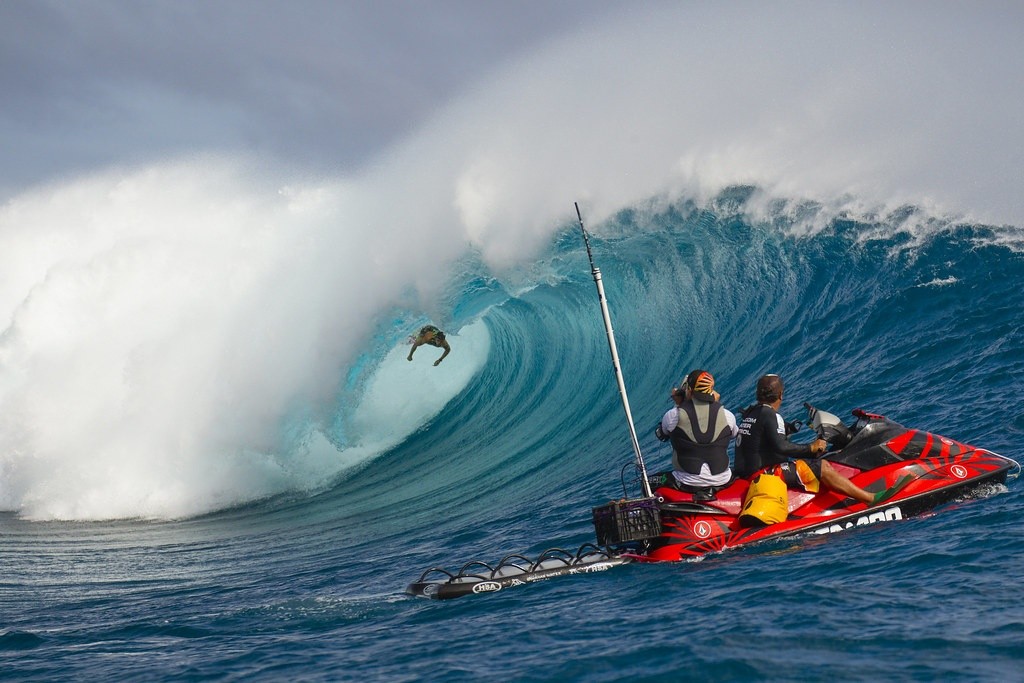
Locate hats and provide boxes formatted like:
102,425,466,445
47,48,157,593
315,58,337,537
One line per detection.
688,370,715,402
757,373,783,395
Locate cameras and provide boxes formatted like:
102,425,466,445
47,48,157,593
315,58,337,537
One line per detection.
676,375,688,397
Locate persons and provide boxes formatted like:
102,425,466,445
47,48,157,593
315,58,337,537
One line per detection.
407,325,451,366
655,370,739,494
733,374,893,507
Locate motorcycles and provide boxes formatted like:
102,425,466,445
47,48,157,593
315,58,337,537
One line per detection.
402,400,1022,600
